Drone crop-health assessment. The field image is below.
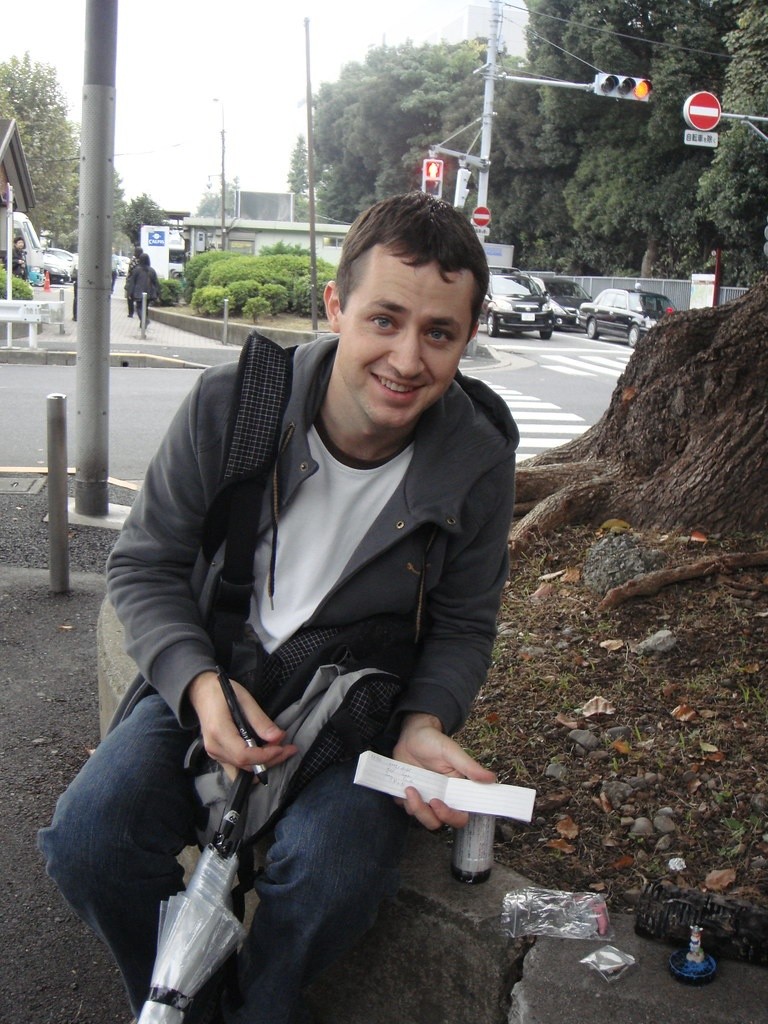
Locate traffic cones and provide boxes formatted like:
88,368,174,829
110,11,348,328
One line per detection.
44,271,50,292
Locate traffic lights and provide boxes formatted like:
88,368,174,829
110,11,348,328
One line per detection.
422,158,442,198
595,73,652,104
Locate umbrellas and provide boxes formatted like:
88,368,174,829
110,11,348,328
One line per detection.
136,734,256,1024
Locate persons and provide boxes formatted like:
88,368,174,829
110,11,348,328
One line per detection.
9,238,161,327
38,191,516,1024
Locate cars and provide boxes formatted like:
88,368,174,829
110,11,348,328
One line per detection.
43,248,131,284
539,276,594,331
577,288,679,347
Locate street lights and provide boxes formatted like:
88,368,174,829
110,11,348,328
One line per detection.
213,96,226,251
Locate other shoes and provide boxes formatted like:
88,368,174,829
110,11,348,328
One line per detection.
141,318,150,329
128,314,133,317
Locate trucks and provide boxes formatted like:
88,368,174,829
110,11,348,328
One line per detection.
0,208,42,284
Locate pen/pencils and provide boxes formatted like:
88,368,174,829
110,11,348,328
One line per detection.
214,663,271,787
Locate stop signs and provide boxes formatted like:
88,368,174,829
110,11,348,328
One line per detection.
684,91,721,132
472,206,491,226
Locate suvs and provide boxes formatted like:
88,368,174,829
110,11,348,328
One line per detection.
478,267,555,341
